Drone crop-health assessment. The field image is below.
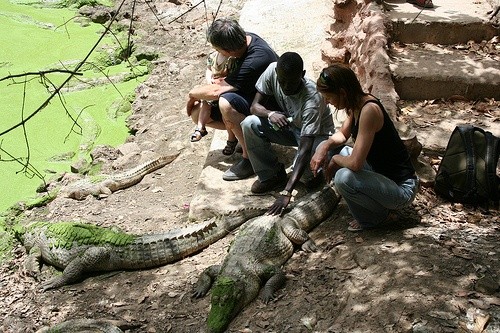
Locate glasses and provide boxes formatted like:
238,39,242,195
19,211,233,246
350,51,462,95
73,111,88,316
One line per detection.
321,68,332,82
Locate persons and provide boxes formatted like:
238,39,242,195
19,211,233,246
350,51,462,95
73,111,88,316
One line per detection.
207,18,280,181
310,65,418,232
191,51,236,156
240,52,335,217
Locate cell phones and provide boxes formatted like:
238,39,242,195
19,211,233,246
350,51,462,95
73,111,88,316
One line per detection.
317,167,322,174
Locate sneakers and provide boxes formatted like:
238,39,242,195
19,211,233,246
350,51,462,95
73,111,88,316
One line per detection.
235,143,243,153
223,156,254,181
307,174,324,189
251,163,287,193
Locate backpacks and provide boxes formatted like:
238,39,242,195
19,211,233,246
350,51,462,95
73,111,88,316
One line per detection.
434,126,500,204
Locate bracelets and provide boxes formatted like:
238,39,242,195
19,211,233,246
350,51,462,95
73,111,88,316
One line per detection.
267,111,278,122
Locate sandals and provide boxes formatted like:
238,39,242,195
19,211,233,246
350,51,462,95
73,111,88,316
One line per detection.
347,216,393,231
222,138,238,156
191,128,208,142
